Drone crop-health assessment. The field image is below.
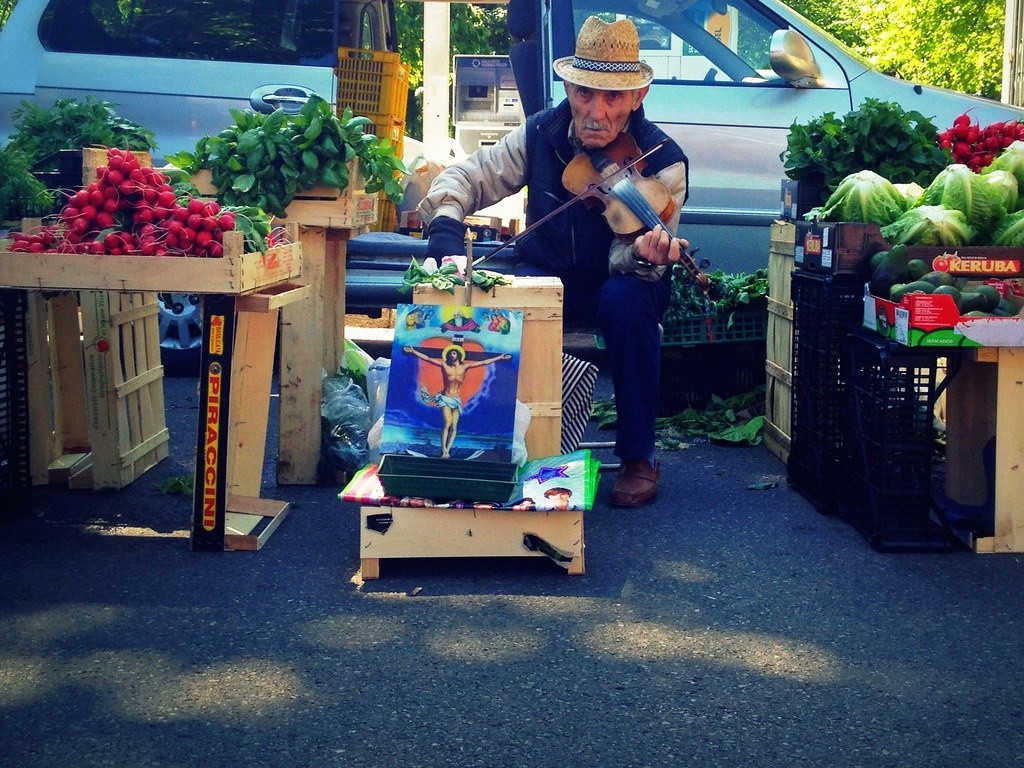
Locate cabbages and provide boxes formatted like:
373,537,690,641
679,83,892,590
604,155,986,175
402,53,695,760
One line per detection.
802,140,1024,246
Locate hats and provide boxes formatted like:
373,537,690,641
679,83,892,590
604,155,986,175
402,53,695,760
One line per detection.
553,15,654,90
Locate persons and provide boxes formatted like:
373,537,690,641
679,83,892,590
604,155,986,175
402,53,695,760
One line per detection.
416,16,690,507
401,487,580,512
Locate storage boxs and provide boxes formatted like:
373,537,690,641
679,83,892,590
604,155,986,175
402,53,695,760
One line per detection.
277,225,346,485
81,147,379,229
334,45,411,239
861,281,1024,347
0,218,303,294
358,500,587,580
0,286,36,514
411,273,564,463
762,224,796,462
780,178,836,222
0,148,82,219
188,283,292,550
399,211,520,248
835,341,955,553
657,347,761,417
796,222,1024,278
787,270,868,517
946,346,1024,554
375,454,520,502
593,301,768,350
26,289,90,487
69,290,171,491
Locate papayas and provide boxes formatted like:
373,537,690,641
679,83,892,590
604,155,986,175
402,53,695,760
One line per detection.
869,243,1019,317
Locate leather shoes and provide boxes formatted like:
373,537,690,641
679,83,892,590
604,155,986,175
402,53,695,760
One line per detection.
609,457,659,508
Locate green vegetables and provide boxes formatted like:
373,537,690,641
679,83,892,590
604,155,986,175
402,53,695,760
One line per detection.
779,96,956,195
318,367,372,472
397,257,512,295
588,266,770,451
0,94,412,255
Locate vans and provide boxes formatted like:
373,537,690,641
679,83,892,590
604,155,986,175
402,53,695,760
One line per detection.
0,0,1024,381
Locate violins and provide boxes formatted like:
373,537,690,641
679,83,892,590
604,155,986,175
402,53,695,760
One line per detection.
561,131,711,290
